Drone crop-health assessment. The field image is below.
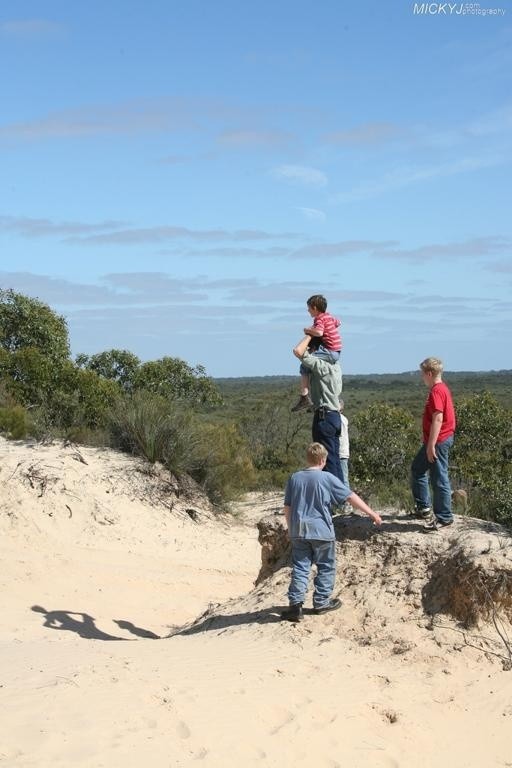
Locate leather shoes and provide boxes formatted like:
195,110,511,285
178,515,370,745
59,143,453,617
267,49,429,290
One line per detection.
316,600,341,614
282,604,303,618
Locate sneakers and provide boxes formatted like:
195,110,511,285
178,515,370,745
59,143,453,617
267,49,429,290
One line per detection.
414,504,432,519
423,519,453,532
292,394,314,412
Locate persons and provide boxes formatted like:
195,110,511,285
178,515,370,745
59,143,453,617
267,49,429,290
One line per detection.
292,335,344,488
277,441,383,621
290,294,343,413
338,413,354,516
411,355,456,534
